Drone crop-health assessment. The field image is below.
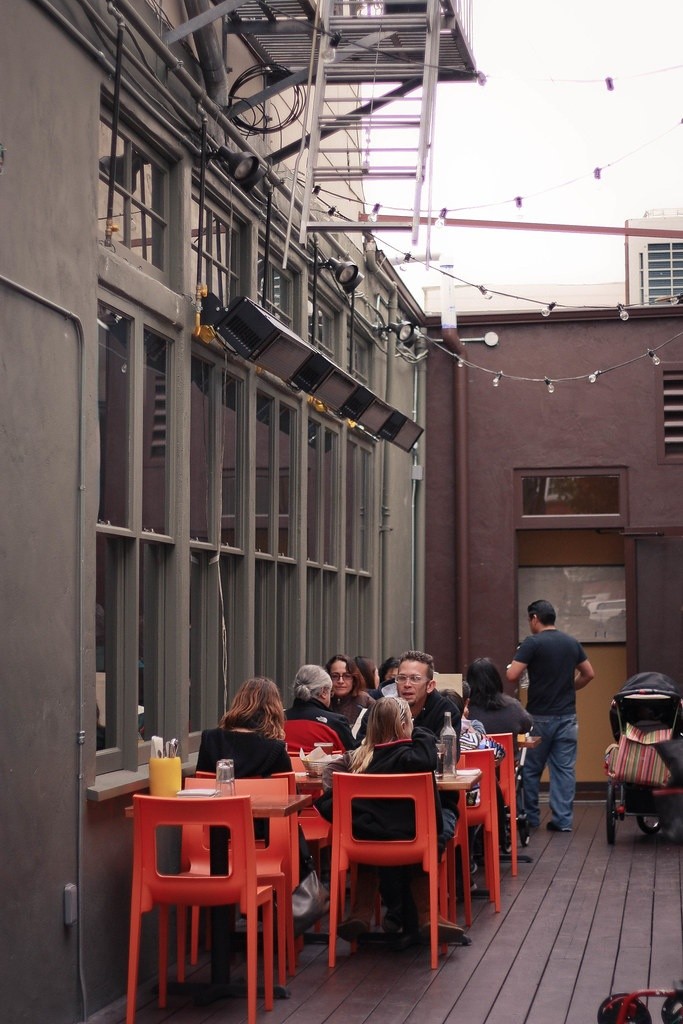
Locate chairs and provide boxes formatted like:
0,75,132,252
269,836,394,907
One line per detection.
124,732,516,1024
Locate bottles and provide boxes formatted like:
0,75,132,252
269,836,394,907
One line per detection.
440,712,456,779
519,668,529,689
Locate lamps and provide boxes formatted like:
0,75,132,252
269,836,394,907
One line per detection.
322,257,359,284
384,320,414,342
291,353,357,412
200,290,315,385
403,333,418,348
342,272,365,296
338,385,393,433
379,411,426,454
217,145,259,181
240,163,268,194
97,151,143,200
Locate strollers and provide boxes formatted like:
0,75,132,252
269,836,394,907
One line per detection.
607,673,683,845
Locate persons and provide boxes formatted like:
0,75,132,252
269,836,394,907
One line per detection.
196,678,309,938
280,650,533,893
96,703,145,750
505,600,595,834
312,696,464,946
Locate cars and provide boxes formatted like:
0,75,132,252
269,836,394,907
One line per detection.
588,599,626,622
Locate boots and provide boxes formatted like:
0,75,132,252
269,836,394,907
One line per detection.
410,876,464,944
337,871,377,943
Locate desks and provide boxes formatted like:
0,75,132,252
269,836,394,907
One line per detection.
124,794,315,1008
291,770,325,792
431,767,484,793
518,733,542,749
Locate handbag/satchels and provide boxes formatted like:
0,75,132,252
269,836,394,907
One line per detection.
291,857,330,940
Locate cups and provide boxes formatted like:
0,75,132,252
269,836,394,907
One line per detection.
314,743,333,756
215,758,236,797
433,744,445,780
149,757,182,799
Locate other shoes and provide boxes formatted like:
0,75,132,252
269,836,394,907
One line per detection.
547,821,572,832
383,910,401,933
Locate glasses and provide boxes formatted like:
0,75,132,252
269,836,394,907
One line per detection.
395,675,424,685
330,672,353,680
